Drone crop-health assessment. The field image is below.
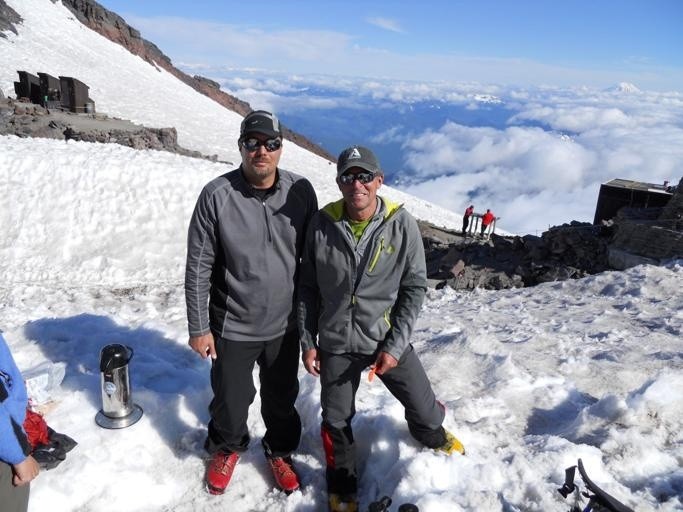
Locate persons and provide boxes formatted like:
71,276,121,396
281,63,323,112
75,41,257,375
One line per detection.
0,334,41,511
184,109,320,495
480,209,494,238
297,144,465,511
461,204,474,235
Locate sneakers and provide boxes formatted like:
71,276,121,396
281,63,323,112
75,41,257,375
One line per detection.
206,450,239,495
434,429,465,456
329,494,358,511
268,457,300,492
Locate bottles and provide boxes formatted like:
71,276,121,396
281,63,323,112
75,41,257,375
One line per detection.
97,343,134,418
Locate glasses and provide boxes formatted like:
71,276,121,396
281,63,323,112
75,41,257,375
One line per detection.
239,137,280,152
340,171,374,184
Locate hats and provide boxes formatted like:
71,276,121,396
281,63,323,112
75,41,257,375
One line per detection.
238,110,282,139
336,147,378,174
